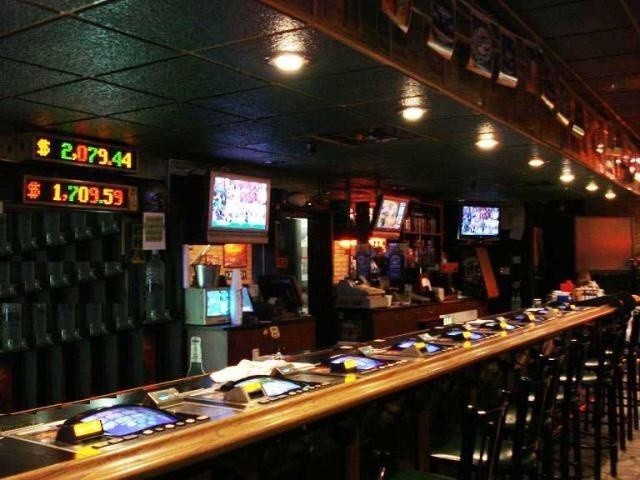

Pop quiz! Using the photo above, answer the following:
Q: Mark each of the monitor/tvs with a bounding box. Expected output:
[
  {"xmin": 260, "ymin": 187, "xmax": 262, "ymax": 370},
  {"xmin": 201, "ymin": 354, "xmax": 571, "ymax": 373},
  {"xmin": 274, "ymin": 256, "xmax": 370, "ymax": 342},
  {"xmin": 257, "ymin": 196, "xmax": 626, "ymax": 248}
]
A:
[
  {"xmin": 73, "ymin": 400, "xmax": 196, "ymax": 438},
  {"xmin": 328, "ymin": 352, "xmax": 391, "ymax": 372},
  {"xmin": 394, "ymin": 336, "xmax": 442, "ymax": 356},
  {"xmin": 233, "ymin": 375, "xmax": 303, "ymax": 398},
  {"xmin": 371, "ymin": 194, "xmax": 409, "ymax": 241},
  {"xmin": 256, "ymin": 275, "xmax": 303, "ymax": 310},
  {"xmin": 478, "ymin": 305, "xmax": 570, "ymax": 333},
  {"xmin": 445, "ymin": 326, "xmax": 488, "ymax": 341},
  {"xmin": 460, "ymin": 202, "xmax": 501, "ymax": 239},
  {"xmin": 206, "ymin": 169, "xmax": 273, "ymax": 244}
]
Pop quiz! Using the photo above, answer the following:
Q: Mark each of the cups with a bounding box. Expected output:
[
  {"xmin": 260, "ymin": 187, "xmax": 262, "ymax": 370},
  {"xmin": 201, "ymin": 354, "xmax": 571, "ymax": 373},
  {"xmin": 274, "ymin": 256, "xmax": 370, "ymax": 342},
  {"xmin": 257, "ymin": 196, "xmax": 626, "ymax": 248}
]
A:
[
  {"xmin": 533, "ymin": 299, "xmax": 542, "ymax": 306},
  {"xmin": 384, "ymin": 288, "xmax": 444, "ymax": 306},
  {"xmin": 229, "ymin": 268, "xmax": 243, "ymax": 325},
  {"xmin": 551, "ymin": 285, "xmax": 604, "ymax": 306}
]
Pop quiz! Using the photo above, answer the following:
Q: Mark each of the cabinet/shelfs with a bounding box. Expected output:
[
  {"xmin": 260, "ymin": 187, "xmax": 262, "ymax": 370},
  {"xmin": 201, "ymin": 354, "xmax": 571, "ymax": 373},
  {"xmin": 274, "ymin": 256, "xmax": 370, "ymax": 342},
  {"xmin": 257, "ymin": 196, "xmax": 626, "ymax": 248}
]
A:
[{"xmin": 307, "ymin": 206, "xmax": 526, "ymax": 339}]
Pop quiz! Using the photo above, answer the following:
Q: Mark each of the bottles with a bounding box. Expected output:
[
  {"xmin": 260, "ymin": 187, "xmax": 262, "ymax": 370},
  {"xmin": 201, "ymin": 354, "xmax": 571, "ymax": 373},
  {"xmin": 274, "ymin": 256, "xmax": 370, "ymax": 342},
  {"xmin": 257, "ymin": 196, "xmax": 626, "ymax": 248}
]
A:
[
  {"xmin": 510, "ymin": 292, "xmax": 522, "ymax": 310},
  {"xmin": 145, "ymin": 249, "xmax": 167, "ymax": 322},
  {"xmin": 186, "ymin": 336, "xmax": 205, "ymax": 378},
  {"xmin": 374, "ymin": 213, "xmax": 437, "ymax": 283}
]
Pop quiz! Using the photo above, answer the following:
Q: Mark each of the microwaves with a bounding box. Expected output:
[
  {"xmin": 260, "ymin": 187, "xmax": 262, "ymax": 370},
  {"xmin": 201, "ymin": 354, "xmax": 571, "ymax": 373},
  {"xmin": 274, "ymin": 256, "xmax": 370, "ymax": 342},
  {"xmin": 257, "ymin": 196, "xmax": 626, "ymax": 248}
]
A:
[{"xmin": 183, "ymin": 287, "xmax": 229, "ymax": 326}]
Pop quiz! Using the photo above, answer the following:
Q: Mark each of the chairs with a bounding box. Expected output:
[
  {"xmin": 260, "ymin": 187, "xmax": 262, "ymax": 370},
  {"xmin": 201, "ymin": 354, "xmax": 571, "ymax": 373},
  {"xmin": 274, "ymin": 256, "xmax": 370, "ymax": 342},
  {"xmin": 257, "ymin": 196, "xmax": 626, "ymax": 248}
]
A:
[{"xmin": 427, "ymin": 305, "xmax": 640, "ymax": 480}]
